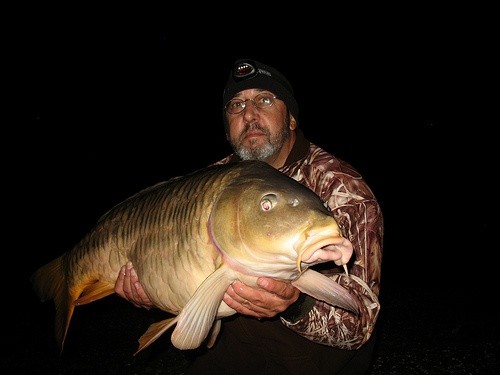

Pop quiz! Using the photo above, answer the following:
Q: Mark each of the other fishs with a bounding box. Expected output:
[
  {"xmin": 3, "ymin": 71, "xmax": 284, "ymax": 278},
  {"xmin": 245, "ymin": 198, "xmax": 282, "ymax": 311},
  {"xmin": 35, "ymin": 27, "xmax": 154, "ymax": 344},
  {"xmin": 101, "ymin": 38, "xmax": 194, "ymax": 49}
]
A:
[{"xmin": 36, "ymin": 158, "xmax": 363, "ymax": 353}]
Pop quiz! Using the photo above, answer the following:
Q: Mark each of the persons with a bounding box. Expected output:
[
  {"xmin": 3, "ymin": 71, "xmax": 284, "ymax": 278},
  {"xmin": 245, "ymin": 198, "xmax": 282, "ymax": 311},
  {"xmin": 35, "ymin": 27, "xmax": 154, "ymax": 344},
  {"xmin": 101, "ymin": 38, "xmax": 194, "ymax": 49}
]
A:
[{"xmin": 114, "ymin": 58, "xmax": 382, "ymax": 375}]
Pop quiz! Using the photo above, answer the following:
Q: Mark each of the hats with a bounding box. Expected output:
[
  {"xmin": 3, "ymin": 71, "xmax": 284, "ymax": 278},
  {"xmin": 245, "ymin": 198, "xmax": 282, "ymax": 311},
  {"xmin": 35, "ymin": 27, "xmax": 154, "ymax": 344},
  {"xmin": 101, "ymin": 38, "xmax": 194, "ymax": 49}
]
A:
[{"xmin": 219, "ymin": 60, "xmax": 301, "ymax": 123}]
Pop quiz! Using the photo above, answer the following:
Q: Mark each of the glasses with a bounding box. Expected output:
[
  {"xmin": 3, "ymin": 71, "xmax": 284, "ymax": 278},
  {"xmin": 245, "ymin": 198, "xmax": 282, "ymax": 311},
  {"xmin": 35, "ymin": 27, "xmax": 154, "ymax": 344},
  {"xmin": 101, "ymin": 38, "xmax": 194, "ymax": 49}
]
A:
[{"xmin": 222, "ymin": 92, "xmax": 283, "ymax": 116}]
